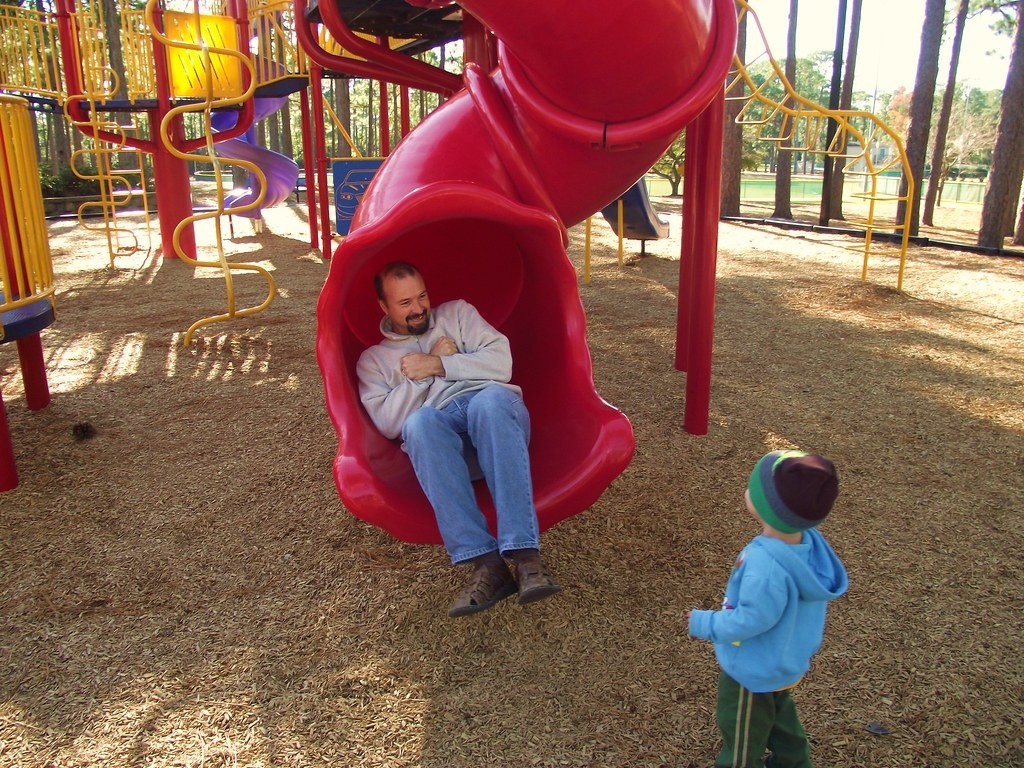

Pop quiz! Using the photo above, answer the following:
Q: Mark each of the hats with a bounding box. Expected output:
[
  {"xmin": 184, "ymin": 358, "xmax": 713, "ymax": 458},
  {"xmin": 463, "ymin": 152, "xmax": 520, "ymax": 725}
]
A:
[{"xmin": 749, "ymin": 449, "xmax": 838, "ymax": 534}]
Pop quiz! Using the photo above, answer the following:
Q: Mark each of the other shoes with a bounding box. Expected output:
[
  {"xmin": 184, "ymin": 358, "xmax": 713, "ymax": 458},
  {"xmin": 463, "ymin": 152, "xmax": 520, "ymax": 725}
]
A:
[{"xmin": 764, "ymin": 754, "xmax": 776, "ymax": 768}]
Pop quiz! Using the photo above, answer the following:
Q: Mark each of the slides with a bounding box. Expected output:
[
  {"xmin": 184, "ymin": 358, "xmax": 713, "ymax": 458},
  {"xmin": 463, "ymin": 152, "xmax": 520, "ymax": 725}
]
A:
[
  {"xmin": 205, "ymin": 0, "xmax": 299, "ymax": 220},
  {"xmin": 602, "ymin": 178, "xmax": 670, "ymax": 239},
  {"xmin": 318, "ymin": 0, "xmax": 736, "ymax": 545}
]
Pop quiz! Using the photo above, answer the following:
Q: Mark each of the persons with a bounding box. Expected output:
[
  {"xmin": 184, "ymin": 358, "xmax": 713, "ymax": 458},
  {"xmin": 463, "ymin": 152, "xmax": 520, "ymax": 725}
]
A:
[
  {"xmin": 356, "ymin": 262, "xmax": 561, "ymax": 616},
  {"xmin": 682, "ymin": 449, "xmax": 849, "ymax": 768}
]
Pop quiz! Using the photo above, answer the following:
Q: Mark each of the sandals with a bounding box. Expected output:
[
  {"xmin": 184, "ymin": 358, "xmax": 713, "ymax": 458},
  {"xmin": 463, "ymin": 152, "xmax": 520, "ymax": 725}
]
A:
[
  {"xmin": 448, "ymin": 548, "xmax": 517, "ymax": 616},
  {"xmin": 517, "ymin": 553, "xmax": 562, "ymax": 604}
]
